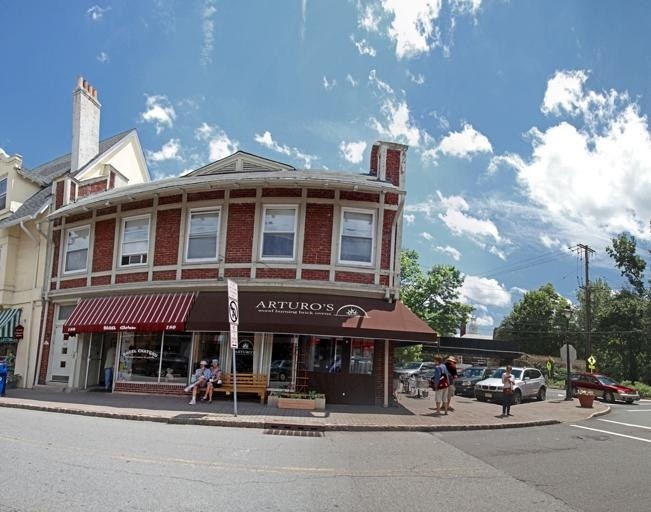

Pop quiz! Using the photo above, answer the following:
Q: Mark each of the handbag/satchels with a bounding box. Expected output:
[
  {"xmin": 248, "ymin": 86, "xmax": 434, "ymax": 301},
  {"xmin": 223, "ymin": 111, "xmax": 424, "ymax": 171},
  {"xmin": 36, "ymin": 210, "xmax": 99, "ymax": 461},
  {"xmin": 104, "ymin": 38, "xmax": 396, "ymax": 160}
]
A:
[{"xmin": 504, "ymin": 387, "xmax": 513, "ymax": 396}]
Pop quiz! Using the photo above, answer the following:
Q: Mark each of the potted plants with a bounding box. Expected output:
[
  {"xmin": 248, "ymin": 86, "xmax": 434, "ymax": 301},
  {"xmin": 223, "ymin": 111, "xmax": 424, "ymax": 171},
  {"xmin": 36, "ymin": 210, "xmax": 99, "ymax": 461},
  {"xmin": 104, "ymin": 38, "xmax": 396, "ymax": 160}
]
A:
[
  {"xmin": 3, "ymin": 351, "xmax": 22, "ymax": 388},
  {"xmin": 269, "ymin": 389, "xmax": 326, "ymax": 411},
  {"xmin": 574, "ymin": 388, "xmax": 596, "ymax": 407}
]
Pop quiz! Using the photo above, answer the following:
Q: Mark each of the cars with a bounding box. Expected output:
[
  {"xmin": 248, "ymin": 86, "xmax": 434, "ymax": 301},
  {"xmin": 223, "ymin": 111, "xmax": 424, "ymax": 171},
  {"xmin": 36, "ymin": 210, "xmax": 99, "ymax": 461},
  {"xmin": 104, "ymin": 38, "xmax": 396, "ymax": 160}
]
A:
[
  {"xmin": 391, "ymin": 361, "xmax": 438, "ymax": 384},
  {"xmin": 566, "ymin": 372, "xmax": 641, "ymax": 404},
  {"xmin": 453, "ymin": 366, "xmax": 494, "ymax": 398},
  {"xmin": 270, "ymin": 359, "xmax": 293, "ymax": 382},
  {"xmin": 132, "ymin": 352, "xmax": 189, "ymax": 378},
  {"xmin": 474, "ymin": 365, "xmax": 547, "ymax": 405}
]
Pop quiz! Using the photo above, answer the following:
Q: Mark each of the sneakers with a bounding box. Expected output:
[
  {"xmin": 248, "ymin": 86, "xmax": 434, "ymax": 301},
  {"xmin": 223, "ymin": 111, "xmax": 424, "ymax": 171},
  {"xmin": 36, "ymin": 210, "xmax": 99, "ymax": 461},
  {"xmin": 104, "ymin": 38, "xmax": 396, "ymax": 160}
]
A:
[
  {"xmin": 189, "ymin": 399, "xmax": 196, "ymax": 405},
  {"xmin": 183, "ymin": 387, "xmax": 191, "ymax": 393}
]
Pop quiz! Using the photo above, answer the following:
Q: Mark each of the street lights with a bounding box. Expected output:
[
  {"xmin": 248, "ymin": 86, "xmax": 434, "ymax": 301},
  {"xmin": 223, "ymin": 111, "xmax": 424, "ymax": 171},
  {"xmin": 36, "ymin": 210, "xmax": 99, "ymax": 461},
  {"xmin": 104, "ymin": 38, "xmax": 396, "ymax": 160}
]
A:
[{"xmin": 562, "ymin": 305, "xmax": 573, "ymax": 401}]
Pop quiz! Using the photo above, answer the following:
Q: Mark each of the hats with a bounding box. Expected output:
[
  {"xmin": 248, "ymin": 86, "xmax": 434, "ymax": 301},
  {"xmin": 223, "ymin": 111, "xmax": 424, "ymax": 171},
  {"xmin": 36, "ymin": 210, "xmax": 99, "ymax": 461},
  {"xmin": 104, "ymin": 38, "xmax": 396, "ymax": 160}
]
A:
[
  {"xmin": 199, "ymin": 360, "xmax": 208, "ymax": 365},
  {"xmin": 446, "ymin": 356, "xmax": 457, "ymax": 364}
]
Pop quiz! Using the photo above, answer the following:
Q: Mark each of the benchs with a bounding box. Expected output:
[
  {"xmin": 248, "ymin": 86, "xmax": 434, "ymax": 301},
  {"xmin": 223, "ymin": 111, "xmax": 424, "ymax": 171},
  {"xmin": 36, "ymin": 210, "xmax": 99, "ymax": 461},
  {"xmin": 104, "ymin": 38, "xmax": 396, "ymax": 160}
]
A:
[{"xmin": 196, "ymin": 374, "xmax": 266, "ymax": 404}]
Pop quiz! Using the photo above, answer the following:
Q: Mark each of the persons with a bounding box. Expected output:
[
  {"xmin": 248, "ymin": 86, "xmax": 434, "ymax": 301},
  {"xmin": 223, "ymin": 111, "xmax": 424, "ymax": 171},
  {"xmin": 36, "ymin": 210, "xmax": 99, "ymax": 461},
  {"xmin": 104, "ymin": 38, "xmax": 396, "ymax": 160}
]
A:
[
  {"xmin": 103, "ymin": 340, "xmax": 117, "ymax": 392},
  {"xmin": 498, "ymin": 365, "xmax": 516, "ymax": 416},
  {"xmin": 328, "ymin": 355, "xmax": 341, "ymax": 373},
  {"xmin": 184, "ymin": 359, "xmax": 222, "ymax": 405},
  {"xmin": 428, "ymin": 353, "xmax": 460, "ymax": 415}
]
